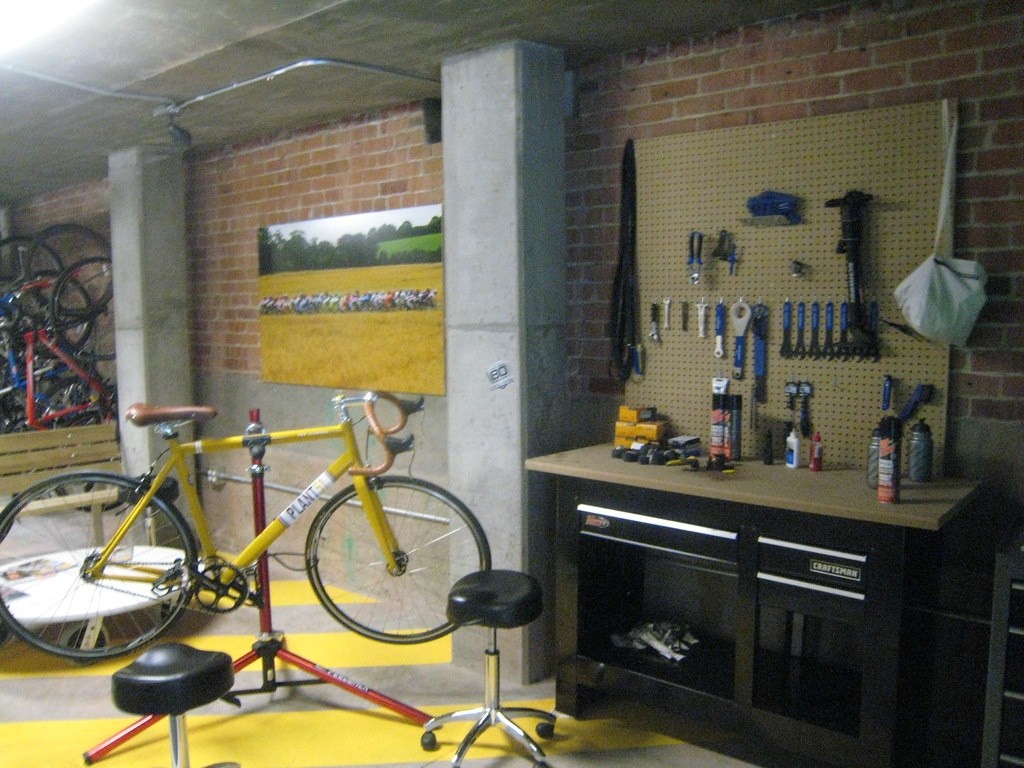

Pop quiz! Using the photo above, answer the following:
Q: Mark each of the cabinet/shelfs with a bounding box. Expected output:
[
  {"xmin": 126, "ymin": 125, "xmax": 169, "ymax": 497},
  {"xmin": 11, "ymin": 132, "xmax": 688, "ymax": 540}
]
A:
[
  {"xmin": 981, "ymin": 530, "xmax": 1024, "ymax": 767},
  {"xmin": 521, "ymin": 435, "xmax": 990, "ymax": 768}
]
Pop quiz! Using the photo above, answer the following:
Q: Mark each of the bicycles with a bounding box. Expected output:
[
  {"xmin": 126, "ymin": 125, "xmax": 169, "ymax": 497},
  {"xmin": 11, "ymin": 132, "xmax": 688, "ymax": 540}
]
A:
[
  {"xmin": 0, "ymin": 390, "xmax": 495, "ymax": 663},
  {"xmin": 0, "ymin": 222, "xmax": 116, "ymax": 437}
]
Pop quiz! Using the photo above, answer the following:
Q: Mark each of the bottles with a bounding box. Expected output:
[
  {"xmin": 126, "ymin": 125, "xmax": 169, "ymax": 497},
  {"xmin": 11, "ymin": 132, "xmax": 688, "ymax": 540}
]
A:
[
  {"xmin": 786, "ymin": 428, "xmax": 800, "ymax": 469},
  {"xmin": 710, "ymin": 377, "xmax": 731, "ymax": 462},
  {"xmin": 867, "ymin": 429, "xmax": 878, "ymax": 489},
  {"xmin": 809, "ymin": 432, "xmax": 824, "ymax": 471},
  {"xmin": 879, "ymin": 415, "xmax": 903, "ymax": 503},
  {"xmin": 731, "ymin": 392, "xmax": 745, "ymax": 459},
  {"xmin": 908, "ymin": 415, "xmax": 932, "ymax": 483}
]
